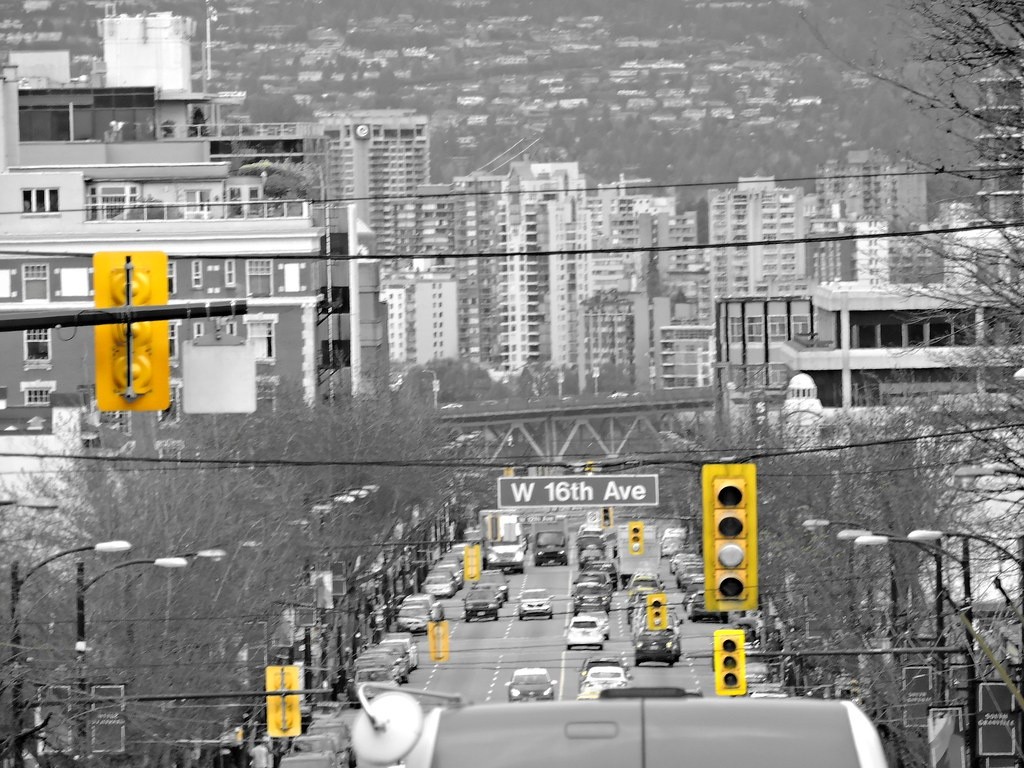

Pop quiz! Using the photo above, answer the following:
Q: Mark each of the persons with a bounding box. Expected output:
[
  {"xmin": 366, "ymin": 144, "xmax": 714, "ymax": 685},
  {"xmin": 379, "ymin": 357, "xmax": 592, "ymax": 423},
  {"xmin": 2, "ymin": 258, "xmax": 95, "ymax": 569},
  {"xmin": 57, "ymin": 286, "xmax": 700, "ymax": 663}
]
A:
[{"xmin": 222, "ymin": 707, "xmax": 312, "ymax": 768}]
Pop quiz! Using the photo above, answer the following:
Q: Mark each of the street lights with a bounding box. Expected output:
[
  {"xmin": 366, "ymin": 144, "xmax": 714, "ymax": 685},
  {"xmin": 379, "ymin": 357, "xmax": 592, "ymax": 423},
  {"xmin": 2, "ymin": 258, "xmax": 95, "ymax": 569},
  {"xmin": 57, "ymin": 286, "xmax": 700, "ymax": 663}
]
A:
[
  {"xmin": 834, "ymin": 527, "xmax": 950, "ymax": 706},
  {"xmin": 7, "ymin": 536, "xmax": 134, "ymax": 768},
  {"xmin": 853, "ymin": 532, "xmax": 981, "ymax": 768},
  {"xmin": 74, "ymin": 553, "xmax": 190, "ymax": 753},
  {"xmin": 904, "ymin": 528, "xmax": 1024, "ymax": 768},
  {"xmin": 163, "ymin": 547, "xmax": 227, "ymax": 639},
  {"xmin": 801, "ymin": 521, "xmax": 901, "ymax": 666},
  {"xmin": 422, "ymin": 370, "xmax": 438, "ymax": 410}
]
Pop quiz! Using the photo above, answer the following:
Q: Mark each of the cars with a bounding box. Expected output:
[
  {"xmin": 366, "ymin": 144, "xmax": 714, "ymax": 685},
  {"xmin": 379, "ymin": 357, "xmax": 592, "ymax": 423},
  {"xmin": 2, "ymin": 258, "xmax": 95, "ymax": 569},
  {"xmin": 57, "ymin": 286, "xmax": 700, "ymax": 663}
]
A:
[
  {"xmin": 515, "ymin": 588, "xmax": 557, "ymax": 621},
  {"xmin": 277, "ymin": 511, "xmax": 731, "ymax": 768},
  {"xmin": 504, "ymin": 667, "xmax": 558, "ymax": 702}
]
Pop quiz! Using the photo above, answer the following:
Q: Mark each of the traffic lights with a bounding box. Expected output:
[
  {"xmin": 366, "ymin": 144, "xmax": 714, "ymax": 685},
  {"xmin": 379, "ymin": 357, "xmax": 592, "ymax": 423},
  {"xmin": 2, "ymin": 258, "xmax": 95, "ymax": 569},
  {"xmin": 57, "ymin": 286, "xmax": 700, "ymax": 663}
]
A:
[
  {"xmin": 645, "ymin": 593, "xmax": 667, "ymax": 631},
  {"xmin": 628, "ymin": 520, "xmax": 645, "ymax": 556},
  {"xmin": 600, "ymin": 506, "xmax": 614, "ymax": 528},
  {"xmin": 713, "ymin": 629, "xmax": 747, "ymax": 697},
  {"xmin": 699, "ymin": 462, "xmax": 759, "ymax": 615}
]
